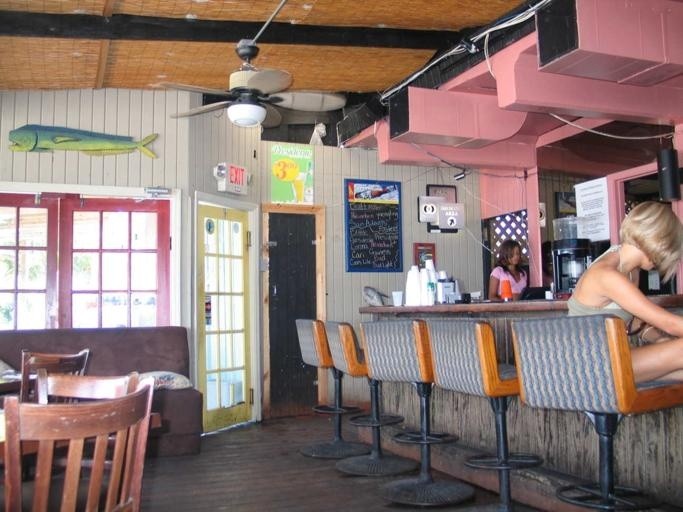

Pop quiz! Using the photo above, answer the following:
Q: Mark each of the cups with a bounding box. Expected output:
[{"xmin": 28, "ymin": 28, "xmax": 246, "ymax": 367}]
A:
[
  {"xmin": 404, "ymin": 259, "xmax": 462, "ymax": 307},
  {"xmin": 391, "ymin": 291, "xmax": 403, "ymax": 307}
]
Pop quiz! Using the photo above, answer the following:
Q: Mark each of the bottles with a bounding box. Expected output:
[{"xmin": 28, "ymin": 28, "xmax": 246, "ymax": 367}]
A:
[
  {"xmin": 354, "ymin": 186, "xmax": 396, "ymax": 201},
  {"xmin": 500, "ymin": 280, "xmax": 512, "ymax": 302}
]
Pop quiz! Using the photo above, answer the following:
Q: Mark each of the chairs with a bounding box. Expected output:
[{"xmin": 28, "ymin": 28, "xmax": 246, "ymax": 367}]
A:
[
  {"xmin": 359, "ymin": 318, "xmax": 476, "ymax": 508},
  {"xmin": 32, "ymin": 367, "xmax": 140, "ymax": 512},
  {"xmin": 323, "ymin": 316, "xmax": 418, "ymax": 477},
  {"xmin": 298, "ymin": 318, "xmax": 372, "ymax": 458},
  {"xmin": 511, "ymin": 312, "xmax": 681, "ymax": 512},
  {"xmin": 0, "ymin": 376, "xmax": 156, "ymax": 512},
  {"xmin": 17, "ymin": 347, "xmax": 88, "ymax": 405},
  {"xmin": 424, "ymin": 317, "xmax": 542, "ymax": 512}
]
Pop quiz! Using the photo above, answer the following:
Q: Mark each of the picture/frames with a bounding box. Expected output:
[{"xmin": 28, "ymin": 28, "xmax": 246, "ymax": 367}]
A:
[
  {"xmin": 425, "ymin": 183, "xmax": 459, "ymax": 234},
  {"xmin": 413, "ymin": 241, "xmax": 436, "ymax": 269}
]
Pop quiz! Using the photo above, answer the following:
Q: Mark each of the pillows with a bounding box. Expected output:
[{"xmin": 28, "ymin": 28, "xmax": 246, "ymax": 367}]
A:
[{"xmin": 140, "ymin": 371, "xmax": 189, "ymax": 390}]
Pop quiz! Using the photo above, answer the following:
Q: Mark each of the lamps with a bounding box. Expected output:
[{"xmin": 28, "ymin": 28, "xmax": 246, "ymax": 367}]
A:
[{"xmin": 222, "ymin": 95, "xmax": 266, "ymax": 126}]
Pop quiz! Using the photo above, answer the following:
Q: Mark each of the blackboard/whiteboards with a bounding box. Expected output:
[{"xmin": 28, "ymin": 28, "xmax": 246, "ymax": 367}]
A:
[{"xmin": 344, "ymin": 178, "xmax": 403, "ymax": 273}]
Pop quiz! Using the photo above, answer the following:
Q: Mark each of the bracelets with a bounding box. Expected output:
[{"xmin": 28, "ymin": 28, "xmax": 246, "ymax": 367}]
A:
[{"xmin": 639, "ymin": 325, "xmax": 655, "ymax": 342}]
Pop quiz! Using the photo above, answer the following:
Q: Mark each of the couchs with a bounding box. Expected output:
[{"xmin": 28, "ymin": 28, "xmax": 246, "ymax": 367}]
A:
[{"xmin": 0, "ymin": 327, "xmax": 204, "ymax": 460}]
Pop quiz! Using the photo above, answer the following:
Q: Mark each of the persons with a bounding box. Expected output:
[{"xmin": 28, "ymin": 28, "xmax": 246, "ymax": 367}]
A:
[
  {"xmin": 568, "ymin": 201, "xmax": 683, "ymax": 383},
  {"xmin": 488, "ymin": 239, "xmax": 527, "ymax": 301}
]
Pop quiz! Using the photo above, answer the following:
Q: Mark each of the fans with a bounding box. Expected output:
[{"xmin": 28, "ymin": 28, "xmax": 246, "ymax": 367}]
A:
[{"xmin": 151, "ymin": 40, "xmax": 345, "ymax": 128}]
